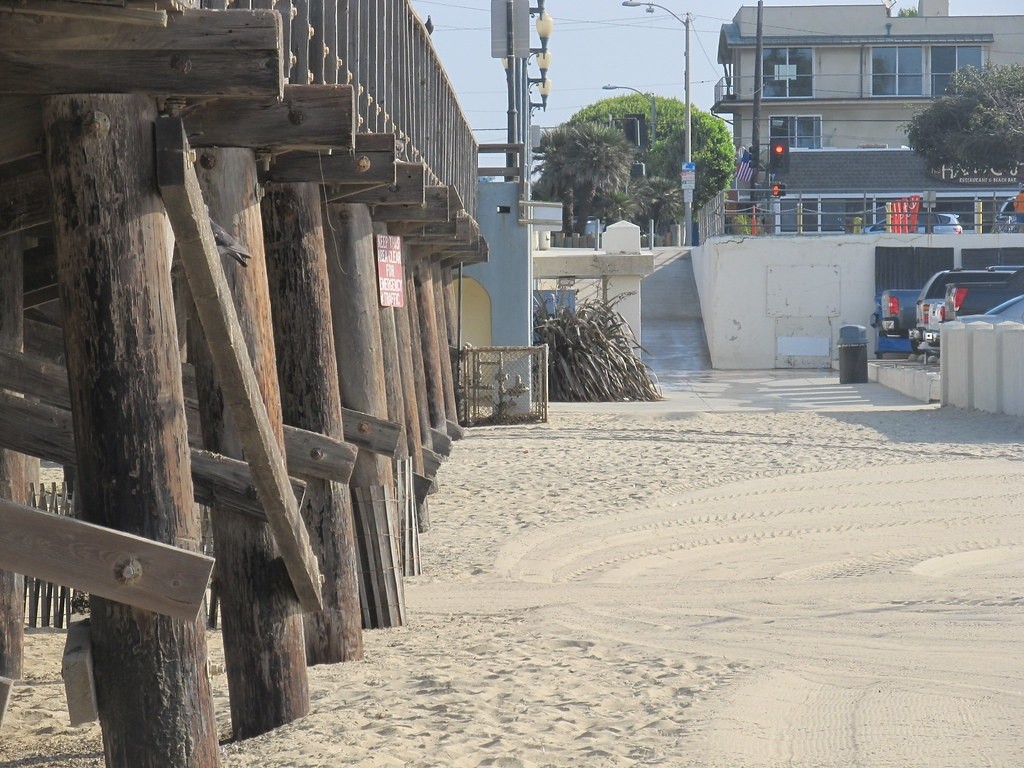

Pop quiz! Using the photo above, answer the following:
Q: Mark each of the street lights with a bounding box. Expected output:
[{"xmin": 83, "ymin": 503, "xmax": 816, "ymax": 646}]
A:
[
  {"xmin": 622, "ymin": 0, "xmax": 693, "ymax": 249},
  {"xmin": 602, "ymin": 85, "xmax": 654, "ymax": 250}
]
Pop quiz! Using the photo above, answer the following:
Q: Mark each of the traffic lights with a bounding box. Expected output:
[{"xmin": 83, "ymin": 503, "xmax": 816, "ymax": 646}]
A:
[
  {"xmin": 773, "ymin": 184, "xmax": 787, "ymax": 198},
  {"xmin": 748, "ymin": 146, "xmax": 760, "ymax": 169},
  {"xmin": 769, "ymin": 140, "xmax": 790, "ymax": 174}
]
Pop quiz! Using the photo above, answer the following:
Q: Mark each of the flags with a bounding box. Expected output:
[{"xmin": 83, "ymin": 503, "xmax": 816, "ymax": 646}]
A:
[{"xmin": 736, "ymin": 150, "xmax": 754, "ymax": 182}]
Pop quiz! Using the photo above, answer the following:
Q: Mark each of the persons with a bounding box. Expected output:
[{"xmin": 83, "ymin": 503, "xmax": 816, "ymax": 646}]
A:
[{"xmin": 1014, "ymin": 186, "xmax": 1024, "ymax": 232}]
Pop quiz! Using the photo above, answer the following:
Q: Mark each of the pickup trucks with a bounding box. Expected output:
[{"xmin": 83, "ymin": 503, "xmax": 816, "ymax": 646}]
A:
[
  {"xmin": 880, "ymin": 290, "xmax": 922, "ymax": 338},
  {"xmin": 943, "ymin": 268, "xmax": 1024, "ymax": 322}
]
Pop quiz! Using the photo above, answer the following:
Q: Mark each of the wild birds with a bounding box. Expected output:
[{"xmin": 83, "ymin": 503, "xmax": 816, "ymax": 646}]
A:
[{"xmin": 425, "ymin": 15, "xmax": 434, "ymax": 35}]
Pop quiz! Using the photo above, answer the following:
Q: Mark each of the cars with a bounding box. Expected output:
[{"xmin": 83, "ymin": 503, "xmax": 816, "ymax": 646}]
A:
[
  {"xmin": 862, "ymin": 212, "xmax": 963, "ymax": 236},
  {"xmin": 908, "ymin": 265, "xmax": 1024, "ymax": 355},
  {"xmin": 994, "ymin": 196, "xmax": 1017, "ymax": 231},
  {"xmin": 952, "ymin": 292, "xmax": 1024, "ymax": 326}
]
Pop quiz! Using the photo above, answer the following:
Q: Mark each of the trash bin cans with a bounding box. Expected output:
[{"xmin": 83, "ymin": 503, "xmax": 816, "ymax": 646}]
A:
[
  {"xmin": 837, "ymin": 325, "xmax": 868, "ymax": 384},
  {"xmin": 666, "ymin": 232, "xmax": 672, "ymax": 246},
  {"xmin": 539, "ymin": 230, "xmax": 550, "ymax": 250},
  {"xmin": 670, "ymin": 225, "xmax": 680, "ymax": 247},
  {"xmin": 842, "ymin": 216, "xmax": 855, "ymax": 234},
  {"xmin": 853, "ymin": 217, "xmax": 862, "ymax": 233},
  {"xmin": 533, "ymin": 231, "xmax": 540, "ymax": 251}
]
